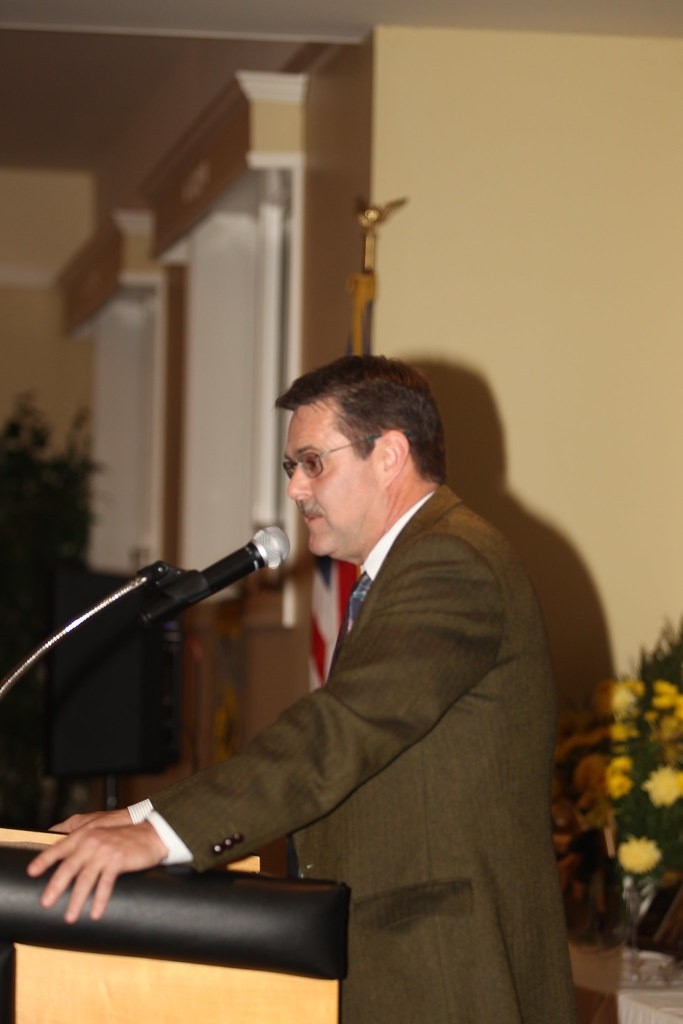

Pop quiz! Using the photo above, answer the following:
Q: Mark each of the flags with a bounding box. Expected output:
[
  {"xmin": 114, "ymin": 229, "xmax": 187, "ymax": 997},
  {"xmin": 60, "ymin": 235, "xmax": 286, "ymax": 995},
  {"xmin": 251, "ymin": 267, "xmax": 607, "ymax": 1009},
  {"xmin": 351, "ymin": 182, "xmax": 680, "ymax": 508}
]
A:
[{"xmin": 313, "ymin": 273, "xmax": 371, "ymax": 690}]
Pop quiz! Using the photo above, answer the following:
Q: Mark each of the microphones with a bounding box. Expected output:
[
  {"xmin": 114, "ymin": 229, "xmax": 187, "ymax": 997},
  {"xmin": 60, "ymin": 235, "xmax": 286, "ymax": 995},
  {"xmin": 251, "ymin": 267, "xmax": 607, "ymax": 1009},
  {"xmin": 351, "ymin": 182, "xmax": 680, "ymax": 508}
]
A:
[{"xmin": 129, "ymin": 525, "xmax": 291, "ymax": 632}]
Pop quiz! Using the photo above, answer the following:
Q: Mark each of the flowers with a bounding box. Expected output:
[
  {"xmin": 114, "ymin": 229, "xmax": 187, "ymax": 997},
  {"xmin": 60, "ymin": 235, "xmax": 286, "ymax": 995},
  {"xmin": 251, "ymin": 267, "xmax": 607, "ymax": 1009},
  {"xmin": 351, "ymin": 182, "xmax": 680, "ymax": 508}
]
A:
[{"xmin": 551, "ymin": 625, "xmax": 683, "ymax": 941}]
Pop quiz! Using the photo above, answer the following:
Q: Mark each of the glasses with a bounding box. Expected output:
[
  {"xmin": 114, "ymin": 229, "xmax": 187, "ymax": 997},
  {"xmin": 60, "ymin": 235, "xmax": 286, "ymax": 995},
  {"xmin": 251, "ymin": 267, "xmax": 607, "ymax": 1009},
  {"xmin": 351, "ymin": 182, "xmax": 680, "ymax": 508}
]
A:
[{"xmin": 282, "ymin": 429, "xmax": 418, "ymax": 480}]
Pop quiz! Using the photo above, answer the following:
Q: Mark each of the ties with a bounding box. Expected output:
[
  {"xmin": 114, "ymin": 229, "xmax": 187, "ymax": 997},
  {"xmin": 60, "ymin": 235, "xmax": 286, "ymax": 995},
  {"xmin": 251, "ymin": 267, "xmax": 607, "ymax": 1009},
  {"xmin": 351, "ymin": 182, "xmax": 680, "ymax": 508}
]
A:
[{"xmin": 343, "ymin": 572, "xmax": 372, "ymax": 636}]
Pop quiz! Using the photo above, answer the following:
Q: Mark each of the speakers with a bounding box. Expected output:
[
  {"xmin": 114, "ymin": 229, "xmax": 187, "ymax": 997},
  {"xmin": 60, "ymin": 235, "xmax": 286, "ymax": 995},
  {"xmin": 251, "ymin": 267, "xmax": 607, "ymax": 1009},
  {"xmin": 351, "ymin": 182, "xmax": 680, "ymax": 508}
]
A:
[{"xmin": 43, "ymin": 571, "xmax": 181, "ymax": 775}]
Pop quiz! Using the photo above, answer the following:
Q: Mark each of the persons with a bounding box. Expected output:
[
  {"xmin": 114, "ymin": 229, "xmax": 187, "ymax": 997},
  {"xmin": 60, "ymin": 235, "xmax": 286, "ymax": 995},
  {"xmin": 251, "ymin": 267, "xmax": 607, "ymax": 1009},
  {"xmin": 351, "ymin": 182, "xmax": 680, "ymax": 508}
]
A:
[{"xmin": 28, "ymin": 355, "xmax": 576, "ymax": 1024}]
